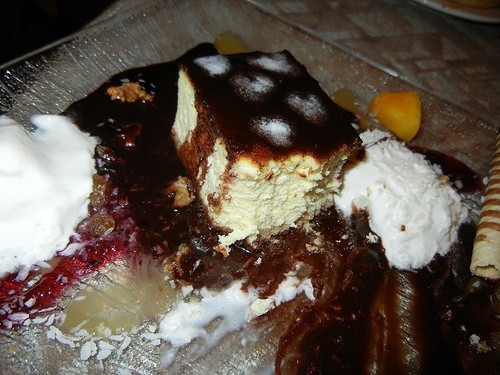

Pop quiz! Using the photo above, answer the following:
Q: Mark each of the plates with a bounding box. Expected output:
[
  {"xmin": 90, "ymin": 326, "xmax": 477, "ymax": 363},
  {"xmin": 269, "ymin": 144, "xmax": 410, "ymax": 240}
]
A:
[{"xmin": 0, "ymin": 0, "xmax": 500, "ymax": 375}]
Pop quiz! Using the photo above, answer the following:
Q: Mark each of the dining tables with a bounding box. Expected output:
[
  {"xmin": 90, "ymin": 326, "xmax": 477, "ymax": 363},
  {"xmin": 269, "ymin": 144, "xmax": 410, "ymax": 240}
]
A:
[{"xmin": 2, "ymin": 2, "xmax": 500, "ymax": 375}]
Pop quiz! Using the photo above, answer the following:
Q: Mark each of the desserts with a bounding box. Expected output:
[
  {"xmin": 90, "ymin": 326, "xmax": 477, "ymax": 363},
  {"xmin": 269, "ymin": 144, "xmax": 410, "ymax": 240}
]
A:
[{"xmin": 1, "ymin": 40, "xmax": 500, "ymax": 374}]
